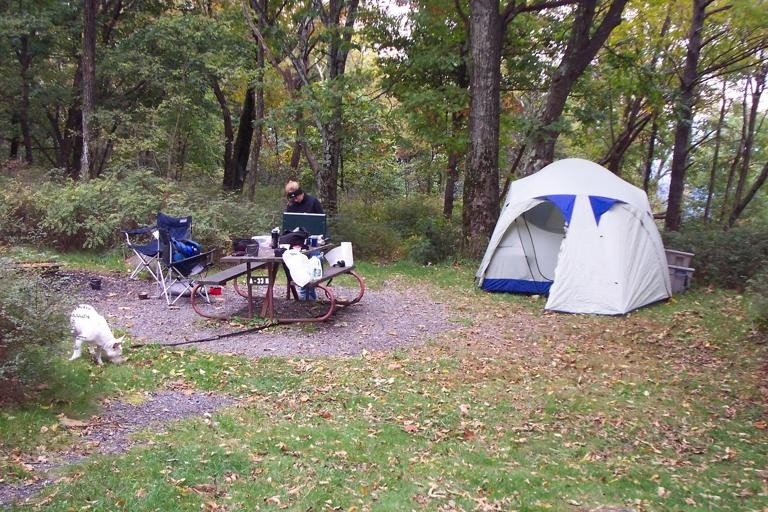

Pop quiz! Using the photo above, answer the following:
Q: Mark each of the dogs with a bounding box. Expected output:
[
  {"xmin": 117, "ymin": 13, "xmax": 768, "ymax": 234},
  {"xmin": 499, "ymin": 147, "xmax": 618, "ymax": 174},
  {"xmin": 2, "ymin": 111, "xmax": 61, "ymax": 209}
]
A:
[{"xmin": 69, "ymin": 304, "xmax": 126, "ymax": 367}]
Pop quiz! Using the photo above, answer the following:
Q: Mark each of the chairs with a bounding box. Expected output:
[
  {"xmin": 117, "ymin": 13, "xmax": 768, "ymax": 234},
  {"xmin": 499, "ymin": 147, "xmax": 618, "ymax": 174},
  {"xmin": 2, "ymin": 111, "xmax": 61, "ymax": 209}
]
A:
[{"xmin": 123, "ymin": 211, "xmax": 216, "ymax": 307}]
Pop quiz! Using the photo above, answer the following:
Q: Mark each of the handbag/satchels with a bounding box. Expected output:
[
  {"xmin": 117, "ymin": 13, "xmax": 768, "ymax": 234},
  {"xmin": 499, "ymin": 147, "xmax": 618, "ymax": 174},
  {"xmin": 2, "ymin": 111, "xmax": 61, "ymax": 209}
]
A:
[
  {"xmin": 278, "ymin": 227, "xmax": 309, "ymax": 251},
  {"xmin": 174, "ymin": 238, "xmax": 201, "ymax": 258},
  {"xmin": 234, "ymin": 238, "xmax": 258, "ymax": 256}
]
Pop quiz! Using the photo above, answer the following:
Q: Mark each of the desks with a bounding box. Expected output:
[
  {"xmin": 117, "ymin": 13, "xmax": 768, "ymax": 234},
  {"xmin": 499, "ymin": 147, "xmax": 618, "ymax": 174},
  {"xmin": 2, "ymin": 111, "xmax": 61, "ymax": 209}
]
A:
[{"xmin": 188, "ymin": 243, "xmax": 365, "ymax": 323}]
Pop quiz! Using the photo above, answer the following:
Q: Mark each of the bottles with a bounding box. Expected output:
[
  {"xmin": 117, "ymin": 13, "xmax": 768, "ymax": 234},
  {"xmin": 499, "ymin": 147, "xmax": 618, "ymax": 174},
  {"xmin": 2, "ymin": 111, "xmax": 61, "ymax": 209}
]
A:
[{"xmin": 271, "ymin": 229, "xmax": 278, "ymax": 249}]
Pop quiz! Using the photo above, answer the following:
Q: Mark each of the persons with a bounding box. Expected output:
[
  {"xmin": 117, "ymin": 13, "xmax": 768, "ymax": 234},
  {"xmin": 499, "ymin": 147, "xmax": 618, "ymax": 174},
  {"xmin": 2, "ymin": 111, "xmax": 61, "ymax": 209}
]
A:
[{"xmin": 284, "ymin": 180, "xmax": 326, "ymax": 302}]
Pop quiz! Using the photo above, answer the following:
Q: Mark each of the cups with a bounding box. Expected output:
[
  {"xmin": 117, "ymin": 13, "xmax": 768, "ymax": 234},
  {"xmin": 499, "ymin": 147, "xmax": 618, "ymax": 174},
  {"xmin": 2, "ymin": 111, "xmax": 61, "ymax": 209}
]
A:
[{"xmin": 308, "ymin": 236, "xmax": 318, "ymax": 247}]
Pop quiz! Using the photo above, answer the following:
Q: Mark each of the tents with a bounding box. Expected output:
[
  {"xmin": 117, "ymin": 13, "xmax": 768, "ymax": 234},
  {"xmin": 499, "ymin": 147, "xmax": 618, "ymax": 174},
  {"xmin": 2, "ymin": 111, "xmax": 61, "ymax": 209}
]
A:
[{"xmin": 473, "ymin": 157, "xmax": 673, "ymax": 316}]
